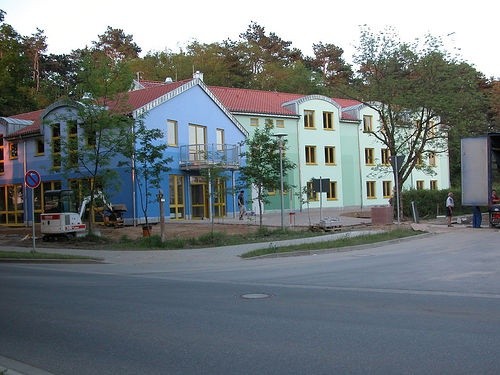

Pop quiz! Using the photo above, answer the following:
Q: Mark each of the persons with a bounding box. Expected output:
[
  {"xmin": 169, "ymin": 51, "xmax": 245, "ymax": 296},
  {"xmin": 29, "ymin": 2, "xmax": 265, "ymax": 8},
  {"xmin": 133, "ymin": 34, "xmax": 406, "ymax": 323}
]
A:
[
  {"xmin": 488, "ymin": 189, "xmax": 500, "ymax": 223},
  {"xmin": 446, "ymin": 192, "xmax": 454, "ymax": 227},
  {"xmin": 237, "ymin": 190, "xmax": 246, "ymax": 220},
  {"xmin": 471, "ymin": 205, "xmax": 483, "ymax": 228}
]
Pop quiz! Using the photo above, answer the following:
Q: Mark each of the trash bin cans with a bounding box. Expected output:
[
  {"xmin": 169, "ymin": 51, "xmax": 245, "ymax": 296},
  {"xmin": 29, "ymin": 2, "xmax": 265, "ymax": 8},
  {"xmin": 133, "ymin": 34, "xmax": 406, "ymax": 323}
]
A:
[{"xmin": 141, "ymin": 225, "xmax": 153, "ymax": 237}]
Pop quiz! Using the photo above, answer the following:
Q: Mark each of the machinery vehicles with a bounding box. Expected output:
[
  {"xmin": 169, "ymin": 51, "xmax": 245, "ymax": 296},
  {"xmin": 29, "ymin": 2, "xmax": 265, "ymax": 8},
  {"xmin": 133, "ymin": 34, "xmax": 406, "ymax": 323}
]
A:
[{"xmin": 40, "ymin": 189, "xmax": 125, "ymax": 243}]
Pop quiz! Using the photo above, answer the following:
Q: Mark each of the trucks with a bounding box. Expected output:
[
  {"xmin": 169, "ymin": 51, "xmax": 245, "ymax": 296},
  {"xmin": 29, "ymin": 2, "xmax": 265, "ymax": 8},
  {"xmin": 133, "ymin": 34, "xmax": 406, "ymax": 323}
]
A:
[{"xmin": 461, "ymin": 132, "xmax": 500, "ymax": 227}]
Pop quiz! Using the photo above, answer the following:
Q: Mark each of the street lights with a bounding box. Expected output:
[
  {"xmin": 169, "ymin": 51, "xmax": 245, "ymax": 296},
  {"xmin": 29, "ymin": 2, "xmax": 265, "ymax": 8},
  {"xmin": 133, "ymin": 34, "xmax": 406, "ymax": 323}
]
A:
[{"xmin": 270, "ymin": 133, "xmax": 288, "ymax": 234}]
